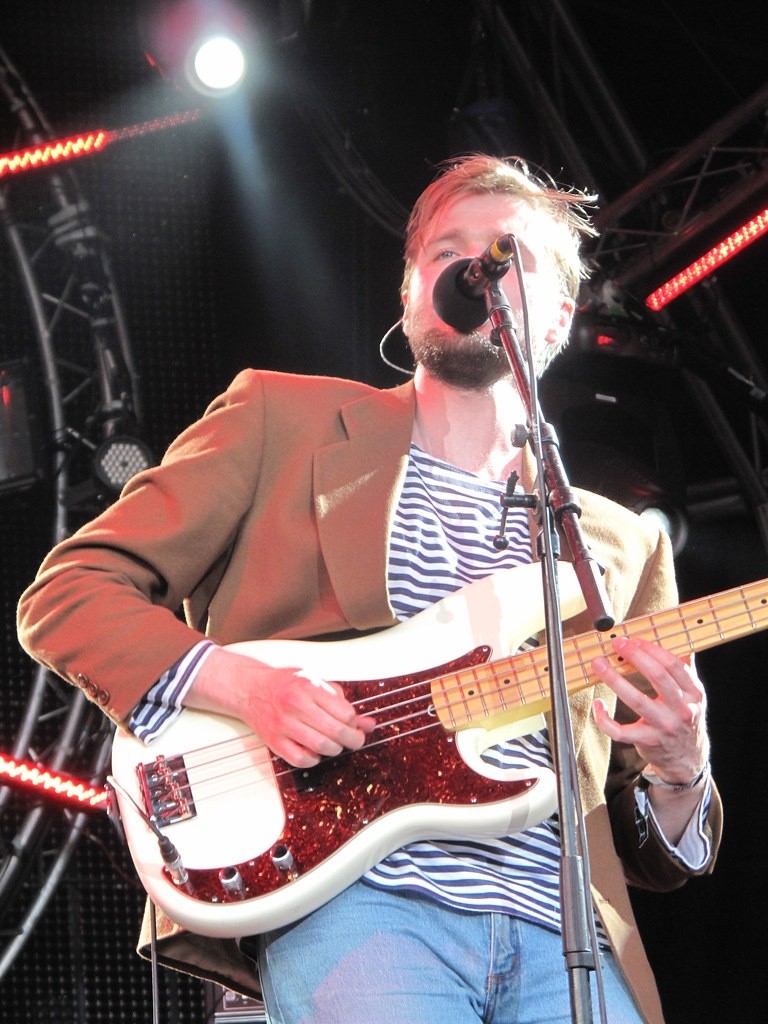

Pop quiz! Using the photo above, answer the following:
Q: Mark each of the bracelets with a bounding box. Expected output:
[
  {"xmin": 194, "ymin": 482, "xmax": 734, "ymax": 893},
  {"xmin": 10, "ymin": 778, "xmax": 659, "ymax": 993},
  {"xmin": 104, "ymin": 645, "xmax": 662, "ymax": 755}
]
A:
[{"xmin": 657, "ymin": 765, "xmax": 706, "ymax": 791}]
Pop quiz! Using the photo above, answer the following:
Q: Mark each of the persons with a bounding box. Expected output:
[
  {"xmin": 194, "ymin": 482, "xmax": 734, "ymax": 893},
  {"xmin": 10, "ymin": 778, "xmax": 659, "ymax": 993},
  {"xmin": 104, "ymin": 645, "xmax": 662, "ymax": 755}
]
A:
[{"xmin": 16, "ymin": 157, "xmax": 724, "ymax": 1024}]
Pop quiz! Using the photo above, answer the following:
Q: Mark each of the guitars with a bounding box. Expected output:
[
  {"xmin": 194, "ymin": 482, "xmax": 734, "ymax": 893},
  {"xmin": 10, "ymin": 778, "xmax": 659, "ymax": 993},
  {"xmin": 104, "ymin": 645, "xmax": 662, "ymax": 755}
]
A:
[{"xmin": 106, "ymin": 557, "xmax": 766, "ymax": 943}]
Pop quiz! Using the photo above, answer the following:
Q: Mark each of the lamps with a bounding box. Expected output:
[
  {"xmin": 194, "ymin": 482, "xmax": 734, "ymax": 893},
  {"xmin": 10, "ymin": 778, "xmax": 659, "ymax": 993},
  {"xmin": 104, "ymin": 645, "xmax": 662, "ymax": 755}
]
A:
[
  {"xmin": 93, "ymin": 434, "xmax": 161, "ymax": 493},
  {"xmin": 622, "ymin": 498, "xmax": 690, "ymax": 560}
]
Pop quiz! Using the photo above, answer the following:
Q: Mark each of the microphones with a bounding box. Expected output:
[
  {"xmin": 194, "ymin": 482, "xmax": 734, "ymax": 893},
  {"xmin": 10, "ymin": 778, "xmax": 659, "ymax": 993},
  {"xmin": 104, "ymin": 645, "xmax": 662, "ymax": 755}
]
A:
[{"xmin": 431, "ymin": 233, "xmax": 518, "ymax": 335}]
